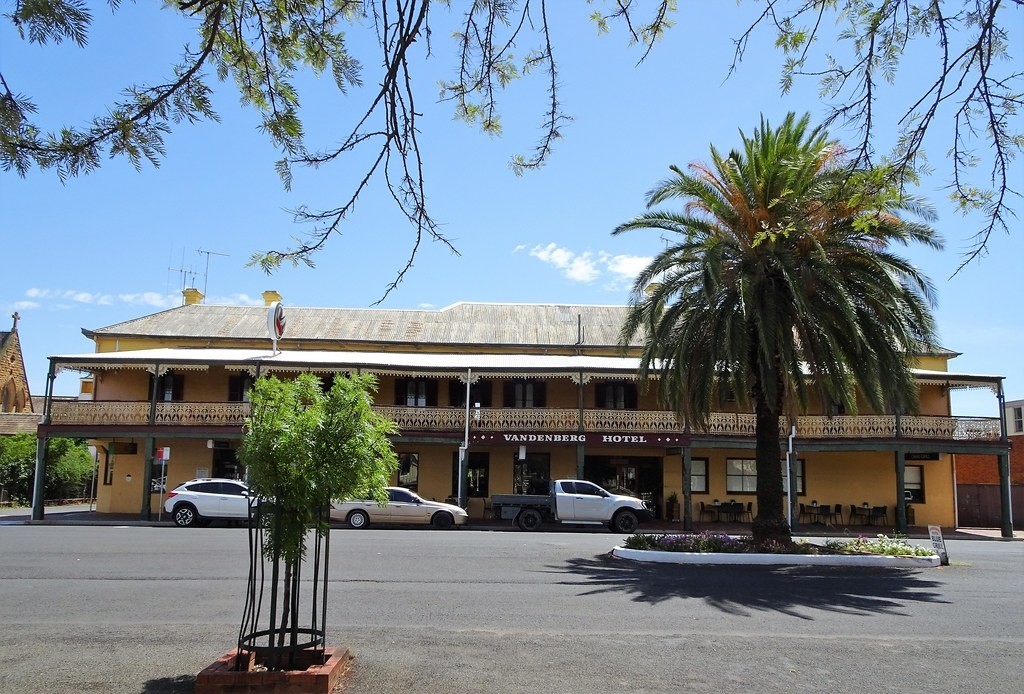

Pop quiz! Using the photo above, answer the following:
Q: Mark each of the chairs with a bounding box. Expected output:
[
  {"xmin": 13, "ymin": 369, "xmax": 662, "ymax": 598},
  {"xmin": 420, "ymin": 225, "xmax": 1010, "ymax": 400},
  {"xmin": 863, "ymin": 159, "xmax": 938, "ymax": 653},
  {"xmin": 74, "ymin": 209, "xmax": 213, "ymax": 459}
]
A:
[
  {"xmin": 849, "ymin": 504, "xmax": 867, "ymax": 528},
  {"xmin": 798, "ymin": 503, "xmax": 816, "ymax": 526},
  {"xmin": 718, "ymin": 503, "xmax": 732, "ymax": 524},
  {"xmin": 740, "ymin": 502, "xmax": 753, "ymax": 523},
  {"xmin": 699, "ymin": 501, "xmax": 715, "ymax": 524},
  {"xmin": 729, "ymin": 503, "xmax": 744, "ymax": 523},
  {"xmin": 867, "ymin": 506, "xmax": 889, "ymax": 528},
  {"xmin": 830, "ymin": 504, "xmax": 843, "ymax": 527},
  {"xmin": 814, "ymin": 504, "xmax": 830, "ymax": 527},
  {"xmin": 481, "ymin": 498, "xmax": 497, "ymax": 520},
  {"xmin": 433, "ymin": 497, "xmax": 470, "ymax": 506}
]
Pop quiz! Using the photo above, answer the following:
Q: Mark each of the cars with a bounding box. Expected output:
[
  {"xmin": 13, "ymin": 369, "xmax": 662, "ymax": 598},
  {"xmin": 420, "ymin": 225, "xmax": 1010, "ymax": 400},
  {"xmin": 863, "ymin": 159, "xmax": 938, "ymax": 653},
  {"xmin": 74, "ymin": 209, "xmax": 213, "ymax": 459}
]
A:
[{"xmin": 330, "ymin": 486, "xmax": 469, "ymax": 530}]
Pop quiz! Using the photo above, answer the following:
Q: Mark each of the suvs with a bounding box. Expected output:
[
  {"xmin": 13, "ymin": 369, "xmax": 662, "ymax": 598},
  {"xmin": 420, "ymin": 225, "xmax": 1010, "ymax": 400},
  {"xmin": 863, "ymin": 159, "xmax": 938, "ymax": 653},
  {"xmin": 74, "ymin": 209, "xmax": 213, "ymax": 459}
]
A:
[{"xmin": 164, "ymin": 477, "xmax": 276, "ymax": 528}]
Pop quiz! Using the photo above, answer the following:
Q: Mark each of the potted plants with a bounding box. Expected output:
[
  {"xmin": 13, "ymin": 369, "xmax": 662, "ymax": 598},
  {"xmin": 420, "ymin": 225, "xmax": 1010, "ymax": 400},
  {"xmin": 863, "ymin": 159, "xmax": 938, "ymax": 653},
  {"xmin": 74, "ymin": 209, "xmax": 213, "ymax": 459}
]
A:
[
  {"xmin": 863, "ymin": 502, "xmax": 869, "ymax": 508},
  {"xmin": 714, "ymin": 499, "xmax": 719, "ymax": 505},
  {"xmin": 448, "ymin": 495, "xmax": 453, "ymax": 499},
  {"xmin": 812, "ymin": 500, "xmax": 817, "ymax": 507},
  {"xmin": 730, "ymin": 499, "xmax": 736, "ymax": 505}
]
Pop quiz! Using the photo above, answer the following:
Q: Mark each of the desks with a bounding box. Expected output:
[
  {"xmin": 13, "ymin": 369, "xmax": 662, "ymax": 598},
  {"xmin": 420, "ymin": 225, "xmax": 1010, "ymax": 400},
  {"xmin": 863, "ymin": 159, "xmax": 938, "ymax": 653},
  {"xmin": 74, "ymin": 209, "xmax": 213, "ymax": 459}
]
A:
[
  {"xmin": 708, "ymin": 504, "xmax": 734, "ymax": 523},
  {"xmin": 443, "ymin": 501, "xmax": 458, "ymax": 503},
  {"xmin": 857, "ymin": 507, "xmax": 874, "ymax": 527},
  {"xmin": 806, "ymin": 505, "xmax": 824, "ymax": 526}
]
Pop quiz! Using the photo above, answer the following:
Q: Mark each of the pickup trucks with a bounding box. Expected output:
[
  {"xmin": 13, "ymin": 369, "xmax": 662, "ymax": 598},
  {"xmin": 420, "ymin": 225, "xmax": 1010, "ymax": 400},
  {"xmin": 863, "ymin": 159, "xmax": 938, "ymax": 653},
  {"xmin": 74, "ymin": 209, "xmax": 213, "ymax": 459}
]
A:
[{"xmin": 491, "ymin": 479, "xmax": 655, "ymax": 534}]
